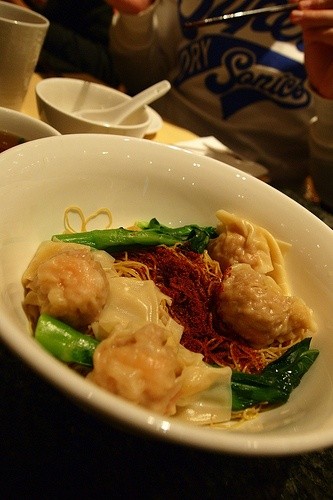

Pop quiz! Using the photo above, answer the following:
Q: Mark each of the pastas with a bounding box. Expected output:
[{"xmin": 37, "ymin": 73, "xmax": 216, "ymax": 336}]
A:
[{"xmin": 62, "ymin": 207, "xmax": 309, "ymax": 431}]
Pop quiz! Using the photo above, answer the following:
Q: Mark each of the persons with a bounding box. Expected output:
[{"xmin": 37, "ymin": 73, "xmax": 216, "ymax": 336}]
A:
[{"xmin": 107, "ymin": 0, "xmax": 333, "ymax": 211}]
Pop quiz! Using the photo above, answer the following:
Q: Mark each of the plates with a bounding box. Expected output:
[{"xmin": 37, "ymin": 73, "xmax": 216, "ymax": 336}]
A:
[{"xmin": 145, "ymin": 104, "xmax": 162, "ymax": 136}]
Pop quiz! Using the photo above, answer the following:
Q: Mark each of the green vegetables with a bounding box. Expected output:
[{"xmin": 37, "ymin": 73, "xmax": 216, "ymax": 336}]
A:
[
  {"xmin": 32, "ymin": 313, "xmax": 319, "ymax": 408},
  {"xmin": 52, "ymin": 216, "xmax": 218, "ymax": 254}
]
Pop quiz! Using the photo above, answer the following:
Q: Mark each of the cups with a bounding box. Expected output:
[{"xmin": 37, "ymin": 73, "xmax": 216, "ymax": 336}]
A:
[{"xmin": 0, "ymin": 0, "xmax": 50, "ymax": 113}]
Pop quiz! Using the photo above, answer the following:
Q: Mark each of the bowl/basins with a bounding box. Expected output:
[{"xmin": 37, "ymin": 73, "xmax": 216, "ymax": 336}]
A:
[
  {"xmin": 35, "ymin": 76, "xmax": 150, "ymax": 139},
  {"xmin": 0, "ymin": 107, "xmax": 62, "ymax": 152},
  {"xmin": 0, "ymin": 133, "xmax": 333, "ymax": 457}
]
